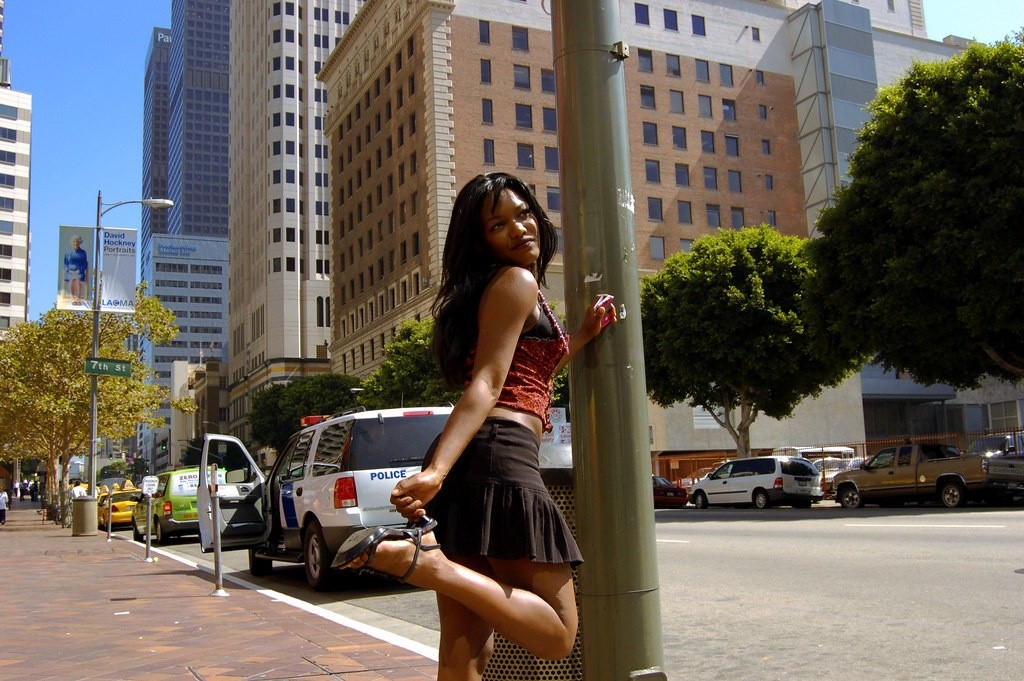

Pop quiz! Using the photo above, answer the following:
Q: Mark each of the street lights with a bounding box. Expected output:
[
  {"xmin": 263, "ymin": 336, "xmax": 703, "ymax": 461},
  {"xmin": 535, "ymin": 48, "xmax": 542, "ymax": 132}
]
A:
[{"xmin": 87, "ymin": 190, "xmax": 175, "ymax": 499}]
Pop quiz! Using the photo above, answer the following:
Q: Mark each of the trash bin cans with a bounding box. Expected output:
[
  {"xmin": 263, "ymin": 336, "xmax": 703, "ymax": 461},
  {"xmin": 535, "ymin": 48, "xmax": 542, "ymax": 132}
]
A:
[{"xmin": 72, "ymin": 495, "xmax": 99, "ymax": 536}]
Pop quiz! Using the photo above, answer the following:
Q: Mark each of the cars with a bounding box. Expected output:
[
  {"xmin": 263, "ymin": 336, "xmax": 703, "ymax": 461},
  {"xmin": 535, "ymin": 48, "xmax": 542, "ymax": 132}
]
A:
[
  {"xmin": 69, "ymin": 480, "xmax": 142, "ymax": 528},
  {"xmin": 132, "ymin": 464, "xmax": 229, "ymax": 546},
  {"xmin": 652, "ymin": 475, "xmax": 687, "ymax": 508},
  {"xmin": 672, "ymin": 432, "xmax": 1024, "ymax": 510}
]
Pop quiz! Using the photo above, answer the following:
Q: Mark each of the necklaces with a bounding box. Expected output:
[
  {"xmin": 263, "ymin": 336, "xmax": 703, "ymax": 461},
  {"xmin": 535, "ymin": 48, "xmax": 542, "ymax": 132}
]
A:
[{"xmin": 538, "ymin": 290, "xmax": 569, "ymax": 354}]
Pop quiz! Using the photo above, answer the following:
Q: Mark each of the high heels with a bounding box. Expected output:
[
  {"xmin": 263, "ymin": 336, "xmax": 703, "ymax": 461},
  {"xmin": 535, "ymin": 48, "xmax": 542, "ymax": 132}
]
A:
[{"xmin": 331, "ymin": 494, "xmax": 442, "ymax": 584}]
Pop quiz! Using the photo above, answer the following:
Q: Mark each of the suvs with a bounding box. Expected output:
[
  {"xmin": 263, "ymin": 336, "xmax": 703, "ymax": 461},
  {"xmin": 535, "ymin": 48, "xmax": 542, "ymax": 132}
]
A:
[{"xmin": 195, "ymin": 403, "xmax": 456, "ymax": 590}]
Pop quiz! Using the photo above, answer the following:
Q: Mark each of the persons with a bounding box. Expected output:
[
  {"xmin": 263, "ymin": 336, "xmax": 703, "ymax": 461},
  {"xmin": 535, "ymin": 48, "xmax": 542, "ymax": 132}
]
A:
[
  {"xmin": 12, "ymin": 479, "xmax": 39, "ymax": 502},
  {"xmin": 64, "ymin": 235, "xmax": 89, "ymax": 305},
  {"xmin": 332, "ymin": 171, "xmax": 618, "ymax": 681},
  {"xmin": 0, "ymin": 486, "xmax": 9, "ymax": 525},
  {"xmin": 69, "ymin": 479, "xmax": 88, "ymax": 499}
]
